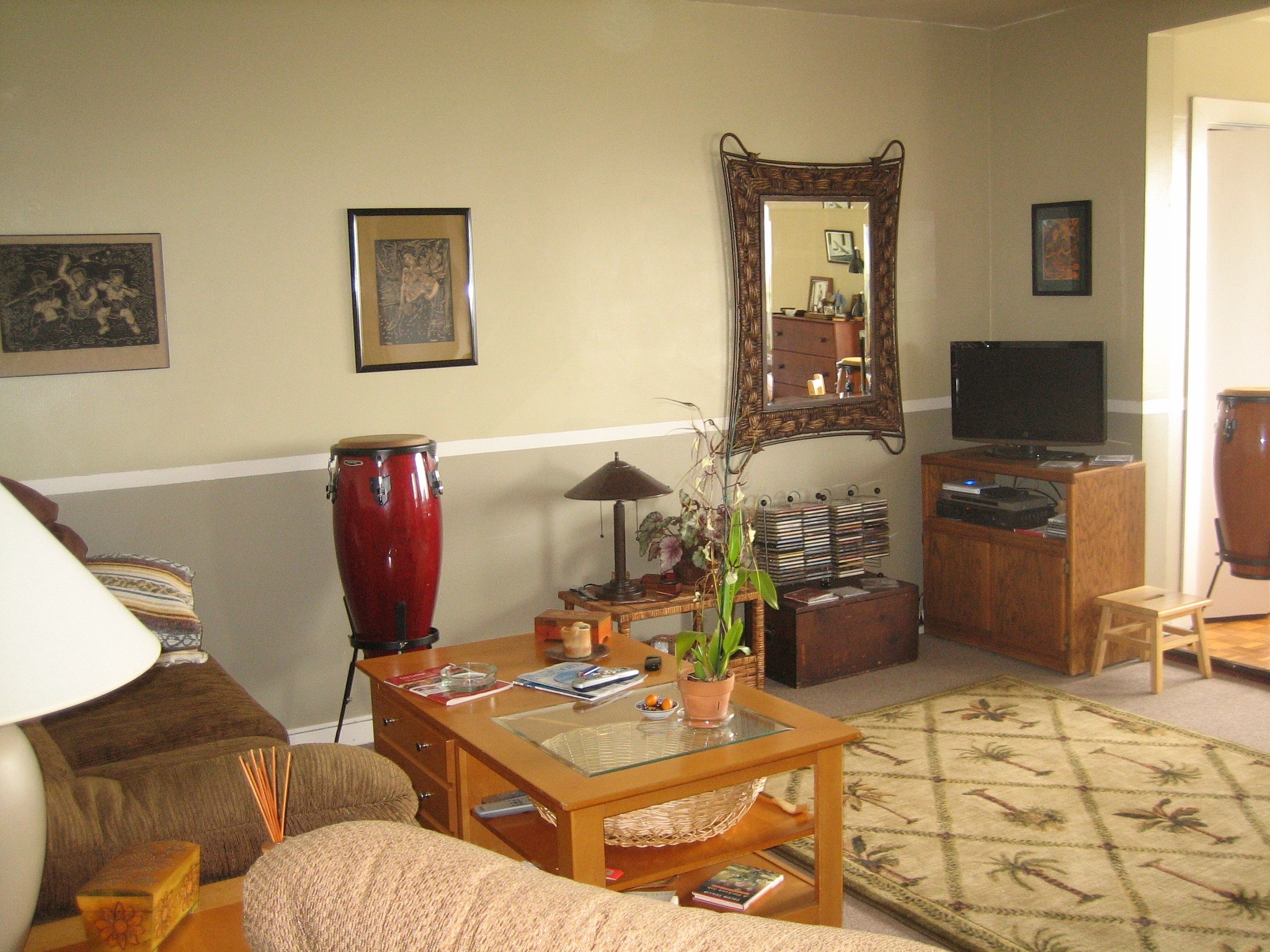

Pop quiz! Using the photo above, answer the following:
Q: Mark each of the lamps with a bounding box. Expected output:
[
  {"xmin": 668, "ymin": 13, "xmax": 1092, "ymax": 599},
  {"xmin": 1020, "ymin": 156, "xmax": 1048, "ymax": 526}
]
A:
[
  {"xmin": 566, "ymin": 453, "xmax": 675, "ymax": 605},
  {"xmin": 847, "ymin": 250, "xmax": 865, "ymax": 275},
  {"xmin": 0, "ymin": 489, "xmax": 161, "ymax": 951}
]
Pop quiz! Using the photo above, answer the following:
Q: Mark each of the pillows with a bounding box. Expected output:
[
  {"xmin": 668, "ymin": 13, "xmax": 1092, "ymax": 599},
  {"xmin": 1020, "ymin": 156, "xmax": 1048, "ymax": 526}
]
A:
[{"xmin": 80, "ymin": 551, "xmax": 208, "ymax": 666}]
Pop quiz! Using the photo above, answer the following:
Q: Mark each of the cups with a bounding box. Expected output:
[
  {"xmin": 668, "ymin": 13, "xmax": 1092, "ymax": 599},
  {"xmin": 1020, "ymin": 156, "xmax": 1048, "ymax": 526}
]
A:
[
  {"xmin": 852, "ymin": 294, "xmax": 864, "ymax": 318},
  {"xmin": 561, "ymin": 622, "xmax": 591, "ymax": 658},
  {"xmin": 644, "ymin": 655, "xmax": 661, "ymax": 671}
]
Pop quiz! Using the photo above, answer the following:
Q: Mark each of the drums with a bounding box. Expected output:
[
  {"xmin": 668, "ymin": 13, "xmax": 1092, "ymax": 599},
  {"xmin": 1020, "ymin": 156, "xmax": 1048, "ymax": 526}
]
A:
[
  {"xmin": 1211, "ymin": 387, "xmax": 1270, "ymax": 581},
  {"xmin": 329, "ymin": 433, "xmax": 444, "ymax": 660}
]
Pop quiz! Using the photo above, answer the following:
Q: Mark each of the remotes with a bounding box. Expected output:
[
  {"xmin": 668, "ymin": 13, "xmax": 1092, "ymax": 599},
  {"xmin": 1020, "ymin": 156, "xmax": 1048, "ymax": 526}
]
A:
[
  {"xmin": 474, "ymin": 789, "xmax": 536, "ymax": 818},
  {"xmin": 571, "ymin": 667, "xmax": 639, "ymax": 693}
]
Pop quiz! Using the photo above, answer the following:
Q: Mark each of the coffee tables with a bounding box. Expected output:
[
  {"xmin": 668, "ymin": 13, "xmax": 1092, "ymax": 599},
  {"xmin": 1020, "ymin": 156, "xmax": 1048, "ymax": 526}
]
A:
[{"xmin": 354, "ymin": 634, "xmax": 859, "ymax": 927}]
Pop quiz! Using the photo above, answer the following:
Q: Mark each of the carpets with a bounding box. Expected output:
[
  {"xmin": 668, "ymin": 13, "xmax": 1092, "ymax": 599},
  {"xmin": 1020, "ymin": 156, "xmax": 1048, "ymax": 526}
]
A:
[{"xmin": 744, "ymin": 676, "xmax": 1270, "ymax": 951}]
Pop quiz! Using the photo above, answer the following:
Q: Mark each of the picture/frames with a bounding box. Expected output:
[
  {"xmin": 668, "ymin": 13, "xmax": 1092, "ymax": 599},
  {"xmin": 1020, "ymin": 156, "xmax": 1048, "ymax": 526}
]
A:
[
  {"xmin": 824, "ymin": 230, "xmax": 854, "ymax": 264},
  {"xmin": 806, "ymin": 276, "xmax": 833, "ymax": 311},
  {"xmin": 346, "ymin": 206, "xmax": 480, "ymax": 373},
  {"xmin": 1030, "ymin": 199, "xmax": 1093, "ymax": 298}
]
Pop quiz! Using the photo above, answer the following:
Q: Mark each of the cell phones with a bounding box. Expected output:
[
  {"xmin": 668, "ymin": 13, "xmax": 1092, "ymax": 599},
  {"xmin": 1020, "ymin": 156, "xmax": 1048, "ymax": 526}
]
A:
[{"xmin": 645, "ymin": 656, "xmax": 661, "ymax": 670}]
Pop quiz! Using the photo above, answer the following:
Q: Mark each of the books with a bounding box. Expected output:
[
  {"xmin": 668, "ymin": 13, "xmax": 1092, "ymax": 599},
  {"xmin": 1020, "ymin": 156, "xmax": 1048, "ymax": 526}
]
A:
[
  {"xmin": 692, "ymin": 865, "xmax": 784, "ymax": 911},
  {"xmin": 383, "ymin": 662, "xmax": 514, "ymax": 706},
  {"xmin": 512, "ymin": 660, "xmax": 648, "ymax": 700}
]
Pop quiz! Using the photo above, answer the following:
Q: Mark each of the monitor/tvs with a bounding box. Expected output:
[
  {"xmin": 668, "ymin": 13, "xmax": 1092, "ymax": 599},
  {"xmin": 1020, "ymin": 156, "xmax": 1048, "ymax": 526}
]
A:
[{"xmin": 950, "ymin": 340, "xmax": 1108, "ymax": 462}]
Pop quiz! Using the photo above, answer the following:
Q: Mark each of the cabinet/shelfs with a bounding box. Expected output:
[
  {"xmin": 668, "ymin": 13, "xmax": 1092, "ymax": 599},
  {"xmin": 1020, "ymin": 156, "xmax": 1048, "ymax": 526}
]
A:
[
  {"xmin": 758, "ymin": 569, "xmax": 922, "ymax": 688},
  {"xmin": 917, "ymin": 444, "xmax": 1144, "ymax": 676},
  {"xmin": 770, "ymin": 312, "xmax": 864, "ymax": 398}
]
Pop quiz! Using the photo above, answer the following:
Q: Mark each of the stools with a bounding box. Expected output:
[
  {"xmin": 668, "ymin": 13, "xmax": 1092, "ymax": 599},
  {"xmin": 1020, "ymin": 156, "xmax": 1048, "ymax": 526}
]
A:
[{"xmin": 1092, "ymin": 586, "xmax": 1212, "ymax": 695}]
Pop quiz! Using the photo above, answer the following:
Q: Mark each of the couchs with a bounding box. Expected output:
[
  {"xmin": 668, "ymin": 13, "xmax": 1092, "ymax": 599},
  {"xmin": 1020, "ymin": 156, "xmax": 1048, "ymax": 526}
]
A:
[{"xmin": 0, "ymin": 477, "xmax": 960, "ymax": 951}]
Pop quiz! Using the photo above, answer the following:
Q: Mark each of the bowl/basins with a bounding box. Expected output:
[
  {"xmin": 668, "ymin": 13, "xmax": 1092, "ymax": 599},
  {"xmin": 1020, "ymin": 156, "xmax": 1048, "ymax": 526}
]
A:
[
  {"xmin": 635, "ymin": 699, "xmax": 679, "ymax": 720},
  {"xmin": 780, "ymin": 307, "xmax": 795, "ymax": 316},
  {"xmin": 785, "ymin": 309, "xmax": 796, "ymax": 316}
]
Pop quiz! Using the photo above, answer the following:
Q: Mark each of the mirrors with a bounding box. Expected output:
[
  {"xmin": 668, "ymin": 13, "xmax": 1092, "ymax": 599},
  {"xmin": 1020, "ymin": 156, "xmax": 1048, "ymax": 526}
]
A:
[{"xmin": 715, "ymin": 131, "xmax": 906, "ymax": 473}]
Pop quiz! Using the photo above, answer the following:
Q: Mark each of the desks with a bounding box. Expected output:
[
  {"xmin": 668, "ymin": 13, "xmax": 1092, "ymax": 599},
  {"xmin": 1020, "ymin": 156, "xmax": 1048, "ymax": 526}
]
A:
[{"xmin": 556, "ymin": 577, "xmax": 767, "ymax": 686}]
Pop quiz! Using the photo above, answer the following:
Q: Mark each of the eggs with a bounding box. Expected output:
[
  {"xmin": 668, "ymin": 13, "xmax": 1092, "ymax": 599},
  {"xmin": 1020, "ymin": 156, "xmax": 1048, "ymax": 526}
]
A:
[
  {"xmin": 661, "ymin": 698, "xmax": 673, "ymax": 711},
  {"xmin": 645, "ymin": 694, "xmax": 658, "ymax": 706}
]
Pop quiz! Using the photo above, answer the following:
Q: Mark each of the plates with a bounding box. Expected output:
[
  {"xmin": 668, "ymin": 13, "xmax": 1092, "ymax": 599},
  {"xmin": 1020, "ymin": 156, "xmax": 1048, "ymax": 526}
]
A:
[{"xmin": 543, "ymin": 644, "xmax": 612, "ymax": 662}]
